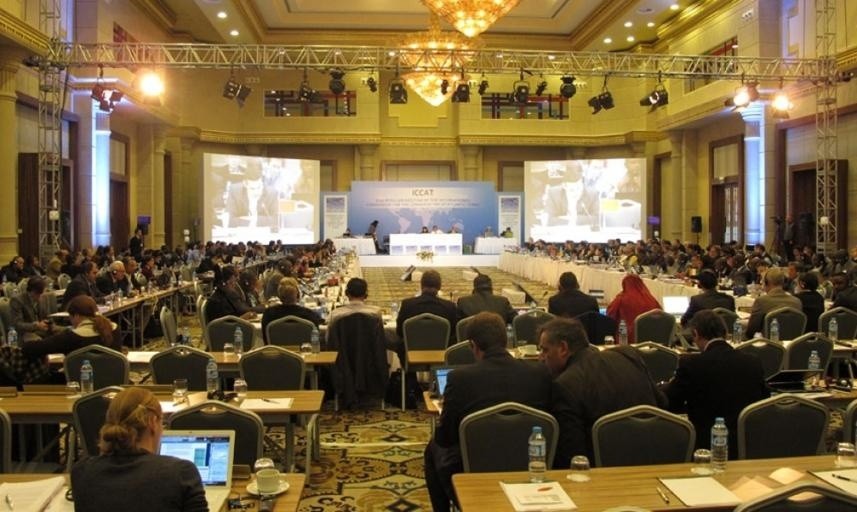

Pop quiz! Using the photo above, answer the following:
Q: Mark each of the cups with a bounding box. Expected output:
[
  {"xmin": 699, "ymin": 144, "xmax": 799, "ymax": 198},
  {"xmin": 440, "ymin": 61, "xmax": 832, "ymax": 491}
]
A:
[
  {"xmin": 567, "ymin": 453, "xmax": 593, "ymax": 482},
  {"xmin": 301, "ymin": 341, "xmax": 311, "ymax": 356},
  {"xmin": 234, "ymin": 377, "xmax": 249, "ymax": 397},
  {"xmin": 692, "ymin": 448, "xmax": 714, "ymax": 477},
  {"xmin": 173, "ymin": 378, "xmax": 188, "ymax": 396},
  {"xmin": 255, "ymin": 468, "xmax": 287, "ymax": 493},
  {"xmin": 830, "ymin": 440, "xmax": 856, "ymax": 467},
  {"xmin": 603, "ymin": 333, "xmax": 616, "ymax": 348},
  {"xmin": 65, "ymin": 381, "xmax": 80, "ymax": 398},
  {"xmin": 223, "ymin": 342, "xmax": 234, "ymax": 355}
]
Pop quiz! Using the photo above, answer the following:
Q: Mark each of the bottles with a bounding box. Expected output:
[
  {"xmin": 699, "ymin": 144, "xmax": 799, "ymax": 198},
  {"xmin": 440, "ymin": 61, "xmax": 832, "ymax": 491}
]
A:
[
  {"xmin": 807, "ymin": 349, "xmax": 820, "ymax": 369},
  {"xmin": 108, "ymin": 260, "xmax": 180, "ymax": 307},
  {"xmin": 505, "ymin": 324, "xmax": 515, "ymax": 348},
  {"xmin": 206, "ymin": 359, "xmax": 219, "ymax": 392},
  {"xmin": 310, "ymin": 326, "xmax": 320, "ymax": 355},
  {"xmin": 527, "ymin": 426, "xmax": 549, "ymax": 484},
  {"xmin": 7, "ymin": 326, "xmax": 18, "ymax": 348},
  {"xmin": 709, "ymin": 417, "xmax": 730, "ymax": 473},
  {"xmin": 233, "ymin": 325, "xmax": 244, "ymax": 355},
  {"xmin": 770, "ymin": 317, "xmax": 781, "ymax": 343},
  {"xmin": 79, "ymin": 359, "xmax": 94, "ymax": 396},
  {"xmin": 732, "ymin": 317, "xmax": 742, "ymax": 343},
  {"xmin": 617, "ymin": 319, "xmax": 628, "ymax": 344},
  {"xmin": 828, "ymin": 316, "xmax": 840, "ymax": 345}
]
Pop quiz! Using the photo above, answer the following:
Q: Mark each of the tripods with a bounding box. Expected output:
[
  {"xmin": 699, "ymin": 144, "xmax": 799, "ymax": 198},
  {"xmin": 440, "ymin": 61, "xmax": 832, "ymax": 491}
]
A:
[{"xmin": 769, "ymin": 227, "xmax": 784, "ymax": 255}]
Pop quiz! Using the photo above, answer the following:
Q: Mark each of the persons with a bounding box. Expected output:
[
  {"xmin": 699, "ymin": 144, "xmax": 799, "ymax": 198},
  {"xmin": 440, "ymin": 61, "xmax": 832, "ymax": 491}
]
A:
[
  {"xmin": 457, "ymin": 273, "xmax": 519, "ymax": 327},
  {"xmin": 424, "ymin": 311, "xmax": 771, "ymax": 512},
  {"xmin": 208, "ymin": 158, "xmax": 318, "ymax": 227},
  {"xmin": 530, "ymin": 160, "xmax": 643, "ymax": 226},
  {"xmin": 524, "ymin": 238, "xmax": 857, "ymax": 382},
  {"xmin": 2, "ymin": 218, "xmax": 382, "ymax": 386},
  {"xmin": 395, "ymin": 268, "xmax": 457, "ymax": 401},
  {"xmin": 421, "ymin": 224, "xmax": 513, "ymax": 237},
  {"xmin": 70, "ymin": 387, "xmax": 210, "ymax": 512}
]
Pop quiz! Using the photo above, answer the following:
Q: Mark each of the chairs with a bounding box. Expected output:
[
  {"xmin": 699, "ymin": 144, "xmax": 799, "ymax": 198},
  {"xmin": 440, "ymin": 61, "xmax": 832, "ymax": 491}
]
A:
[
  {"xmin": 457, "ymin": 400, "xmax": 562, "ymax": 474},
  {"xmin": 731, "ymin": 480, "xmax": 857, "ymax": 511},
  {"xmin": 843, "ymin": 400, "xmax": 857, "ymax": 453},
  {"xmin": 236, "ymin": 344, "xmax": 307, "ymax": 472},
  {"xmin": 167, "ymin": 400, "xmax": 266, "ymax": 469},
  {"xmin": 593, "ymin": 403, "xmax": 699, "ymax": 467},
  {"xmin": 72, "ymin": 386, "xmax": 125, "ymax": 460},
  {"xmin": 738, "ymin": 391, "xmax": 832, "ymax": 460},
  {"xmin": 0, "ymin": 408, "xmax": 15, "ymax": 473}
]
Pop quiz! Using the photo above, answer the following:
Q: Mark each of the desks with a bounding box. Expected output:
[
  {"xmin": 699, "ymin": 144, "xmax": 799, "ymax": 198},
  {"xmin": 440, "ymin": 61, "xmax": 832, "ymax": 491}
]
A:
[
  {"xmin": 499, "ymin": 249, "xmax": 753, "ymax": 315},
  {"xmin": 330, "ymin": 237, "xmax": 377, "ymax": 255},
  {"xmin": 0, "ymin": 470, "xmax": 309, "ymax": 512},
  {"xmin": 0, "ymin": 388, "xmax": 326, "ymax": 489},
  {"xmin": 472, "ymin": 236, "xmax": 518, "ymax": 255},
  {"xmin": 449, "ymin": 452, "xmax": 855, "ymax": 511}
]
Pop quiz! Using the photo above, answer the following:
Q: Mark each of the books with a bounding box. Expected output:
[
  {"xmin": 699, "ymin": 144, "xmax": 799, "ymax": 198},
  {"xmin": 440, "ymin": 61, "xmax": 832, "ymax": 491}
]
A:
[{"xmin": 1, "ymin": 477, "xmax": 66, "ymax": 512}]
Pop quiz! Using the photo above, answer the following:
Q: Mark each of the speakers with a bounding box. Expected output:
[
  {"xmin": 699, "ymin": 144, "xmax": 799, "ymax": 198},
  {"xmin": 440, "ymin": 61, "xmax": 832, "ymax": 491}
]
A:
[
  {"xmin": 691, "ymin": 216, "xmax": 702, "ymax": 233},
  {"xmin": 137, "ymin": 222, "xmax": 148, "ymax": 235}
]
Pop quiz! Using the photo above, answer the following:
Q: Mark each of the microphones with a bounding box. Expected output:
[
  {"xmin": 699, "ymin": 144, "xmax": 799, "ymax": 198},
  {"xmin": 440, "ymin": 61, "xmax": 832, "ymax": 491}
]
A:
[
  {"xmin": 450, "ymin": 292, "xmax": 452, "ymax": 302},
  {"xmin": 536, "ymin": 291, "xmax": 548, "ymax": 307}
]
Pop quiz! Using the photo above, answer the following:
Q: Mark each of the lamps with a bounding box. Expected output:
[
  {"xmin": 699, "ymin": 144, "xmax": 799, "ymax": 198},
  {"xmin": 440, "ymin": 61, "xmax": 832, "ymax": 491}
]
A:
[
  {"xmin": 383, "ymin": 11, "xmax": 485, "ymax": 109},
  {"xmin": 419, "ymin": 0, "xmax": 523, "ymax": 40}
]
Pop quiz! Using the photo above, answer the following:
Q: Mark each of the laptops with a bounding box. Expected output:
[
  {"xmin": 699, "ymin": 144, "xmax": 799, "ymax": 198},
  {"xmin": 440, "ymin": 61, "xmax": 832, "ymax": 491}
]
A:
[
  {"xmin": 599, "ymin": 306, "xmax": 607, "ymax": 316},
  {"xmin": 433, "ymin": 365, "xmax": 458, "ymax": 401},
  {"xmin": 661, "ymin": 295, "xmax": 691, "ymax": 323},
  {"xmin": 159, "ymin": 428, "xmax": 237, "ymax": 512}
]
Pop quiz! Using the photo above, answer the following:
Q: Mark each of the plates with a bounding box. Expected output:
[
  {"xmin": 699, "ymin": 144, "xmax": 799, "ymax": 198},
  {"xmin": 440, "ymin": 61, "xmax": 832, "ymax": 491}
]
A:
[{"xmin": 245, "ymin": 481, "xmax": 290, "ymax": 496}]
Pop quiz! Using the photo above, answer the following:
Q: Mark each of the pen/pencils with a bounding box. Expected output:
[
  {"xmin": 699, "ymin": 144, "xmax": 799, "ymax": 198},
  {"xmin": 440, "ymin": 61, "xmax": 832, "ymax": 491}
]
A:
[
  {"xmin": 656, "ymin": 487, "xmax": 670, "ymax": 503},
  {"xmin": 5, "ymin": 494, "xmax": 15, "ymax": 510},
  {"xmin": 538, "ymin": 485, "xmax": 555, "ymax": 493},
  {"xmin": 831, "ymin": 473, "xmax": 857, "ymax": 484},
  {"xmin": 261, "ymin": 398, "xmax": 279, "ymax": 404}
]
